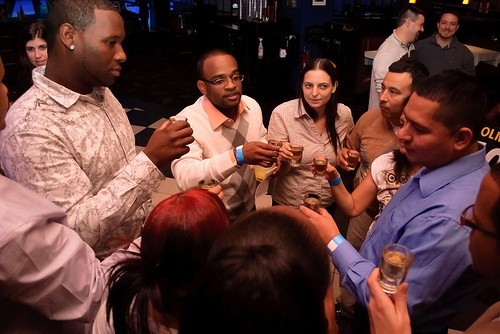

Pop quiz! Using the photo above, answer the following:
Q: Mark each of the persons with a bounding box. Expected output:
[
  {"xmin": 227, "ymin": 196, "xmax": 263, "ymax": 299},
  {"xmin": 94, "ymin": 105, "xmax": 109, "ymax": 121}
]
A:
[
  {"xmin": 0, "ymin": 57, "xmax": 103, "ymax": 334},
  {"xmin": 92, "ymin": 8, "xmax": 500, "ymax": 334},
  {"xmin": 0, "ymin": 0, "xmax": 194, "ymax": 262},
  {"xmin": 23, "ymin": 22, "xmax": 48, "ymax": 68}
]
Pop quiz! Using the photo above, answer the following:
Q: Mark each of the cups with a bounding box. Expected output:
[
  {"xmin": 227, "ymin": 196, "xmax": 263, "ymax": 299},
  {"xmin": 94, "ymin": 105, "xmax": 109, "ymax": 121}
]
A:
[
  {"xmin": 198, "ymin": 178, "xmax": 218, "ymax": 190},
  {"xmin": 169, "ymin": 115, "xmax": 187, "ymax": 125},
  {"xmin": 290, "ymin": 144, "xmax": 304, "ymax": 167},
  {"xmin": 377, "ymin": 244, "xmax": 413, "ymax": 295},
  {"xmin": 314, "ymin": 156, "xmax": 328, "ymax": 174},
  {"xmin": 268, "ymin": 139, "xmax": 283, "ymax": 158},
  {"xmin": 303, "ymin": 193, "xmax": 322, "ymax": 213}
]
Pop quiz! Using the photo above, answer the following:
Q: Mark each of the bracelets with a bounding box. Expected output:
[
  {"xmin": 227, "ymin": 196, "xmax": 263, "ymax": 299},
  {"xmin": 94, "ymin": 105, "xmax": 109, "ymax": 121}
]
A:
[
  {"xmin": 325, "ymin": 233, "xmax": 346, "ymax": 255},
  {"xmin": 329, "ymin": 177, "xmax": 342, "ymax": 186},
  {"xmin": 235, "ymin": 145, "xmax": 244, "ymax": 165}
]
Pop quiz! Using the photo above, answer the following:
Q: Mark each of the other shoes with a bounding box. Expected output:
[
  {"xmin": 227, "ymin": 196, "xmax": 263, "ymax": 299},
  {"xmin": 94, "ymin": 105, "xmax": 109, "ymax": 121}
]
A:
[{"xmin": 334, "ymin": 303, "xmax": 357, "ymax": 320}]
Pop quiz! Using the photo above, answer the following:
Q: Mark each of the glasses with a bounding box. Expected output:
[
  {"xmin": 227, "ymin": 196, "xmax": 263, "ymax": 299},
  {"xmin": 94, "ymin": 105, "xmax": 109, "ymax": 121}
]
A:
[
  {"xmin": 201, "ymin": 75, "xmax": 244, "ymax": 85},
  {"xmin": 460, "ymin": 205, "xmax": 497, "ymax": 238}
]
